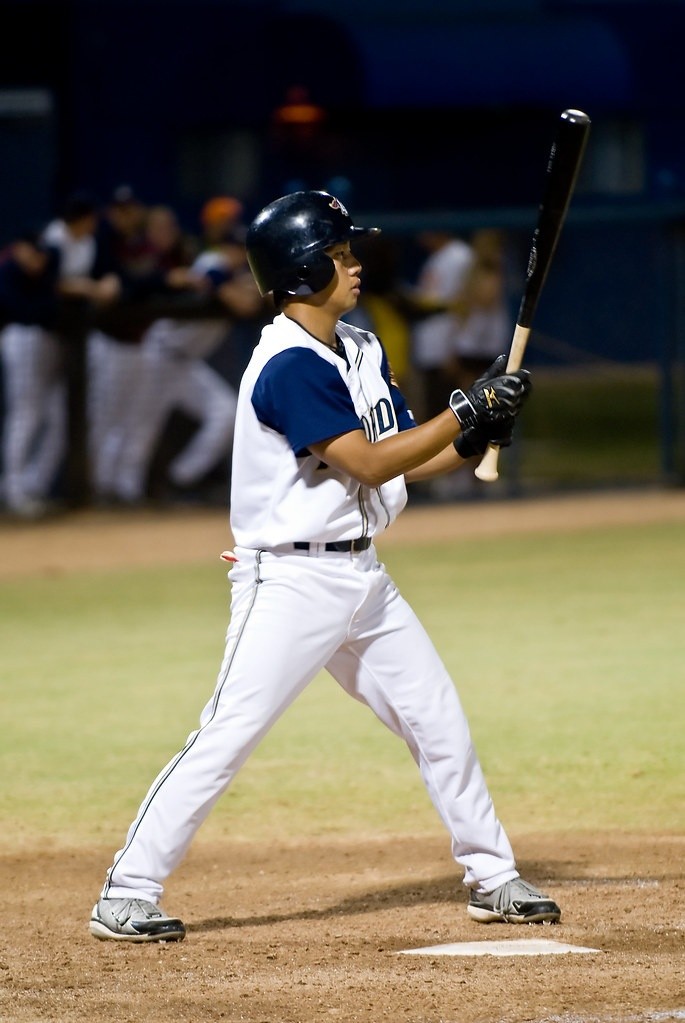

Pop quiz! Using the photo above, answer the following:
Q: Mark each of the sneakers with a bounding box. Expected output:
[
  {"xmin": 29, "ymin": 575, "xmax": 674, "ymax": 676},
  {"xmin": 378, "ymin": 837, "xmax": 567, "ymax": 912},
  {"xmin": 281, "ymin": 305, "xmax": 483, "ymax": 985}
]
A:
[
  {"xmin": 467, "ymin": 877, "xmax": 561, "ymax": 922},
  {"xmin": 89, "ymin": 899, "xmax": 186, "ymax": 941}
]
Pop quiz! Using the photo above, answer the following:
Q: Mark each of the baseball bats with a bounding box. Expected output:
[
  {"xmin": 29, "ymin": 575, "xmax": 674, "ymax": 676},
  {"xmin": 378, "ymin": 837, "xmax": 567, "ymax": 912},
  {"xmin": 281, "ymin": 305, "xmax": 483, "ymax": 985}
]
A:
[{"xmin": 473, "ymin": 109, "xmax": 593, "ymax": 484}]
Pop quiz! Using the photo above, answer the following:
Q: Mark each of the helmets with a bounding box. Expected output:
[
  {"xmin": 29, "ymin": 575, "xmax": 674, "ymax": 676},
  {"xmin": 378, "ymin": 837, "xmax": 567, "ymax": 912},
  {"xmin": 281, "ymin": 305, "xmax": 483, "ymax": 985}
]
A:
[{"xmin": 246, "ymin": 191, "xmax": 382, "ymax": 296}]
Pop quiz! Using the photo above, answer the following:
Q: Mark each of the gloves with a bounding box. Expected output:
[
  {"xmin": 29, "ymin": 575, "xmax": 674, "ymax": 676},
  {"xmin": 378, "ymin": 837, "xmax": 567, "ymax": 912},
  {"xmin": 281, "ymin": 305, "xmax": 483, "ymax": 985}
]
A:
[
  {"xmin": 452, "ymin": 421, "xmax": 513, "ymax": 459},
  {"xmin": 450, "ymin": 353, "xmax": 533, "ymax": 432}
]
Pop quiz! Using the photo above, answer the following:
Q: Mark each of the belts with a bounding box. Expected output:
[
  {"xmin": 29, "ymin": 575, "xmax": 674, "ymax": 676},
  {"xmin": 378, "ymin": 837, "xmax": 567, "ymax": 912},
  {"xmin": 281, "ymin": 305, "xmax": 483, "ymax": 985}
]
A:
[{"xmin": 293, "ymin": 538, "xmax": 372, "ymax": 552}]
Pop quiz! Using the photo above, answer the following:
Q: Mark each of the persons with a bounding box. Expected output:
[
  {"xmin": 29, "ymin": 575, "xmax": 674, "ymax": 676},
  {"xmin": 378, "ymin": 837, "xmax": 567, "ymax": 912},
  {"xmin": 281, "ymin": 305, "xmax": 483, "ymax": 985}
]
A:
[
  {"xmin": 89, "ymin": 189, "xmax": 562, "ymax": 942},
  {"xmin": 0, "ymin": 195, "xmax": 516, "ymax": 521}
]
